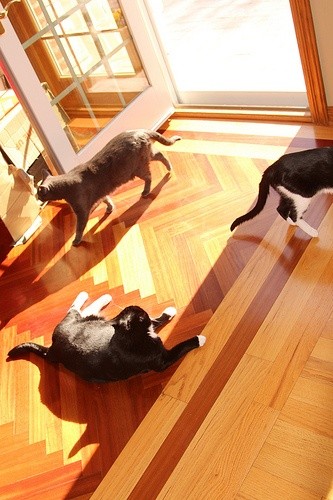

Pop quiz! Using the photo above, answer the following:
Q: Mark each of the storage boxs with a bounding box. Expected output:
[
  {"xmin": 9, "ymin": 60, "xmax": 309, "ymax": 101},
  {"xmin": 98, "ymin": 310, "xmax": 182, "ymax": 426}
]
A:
[{"xmin": 0, "ymin": 82, "xmax": 80, "ymax": 242}]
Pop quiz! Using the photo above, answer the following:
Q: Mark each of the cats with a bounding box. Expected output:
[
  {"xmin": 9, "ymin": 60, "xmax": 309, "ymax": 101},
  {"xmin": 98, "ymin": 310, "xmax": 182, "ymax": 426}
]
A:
[
  {"xmin": 230, "ymin": 147, "xmax": 333, "ymax": 237},
  {"xmin": 35, "ymin": 129, "xmax": 181, "ymax": 246},
  {"xmin": 7, "ymin": 292, "xmax": 206, "ymax": 384}
]
